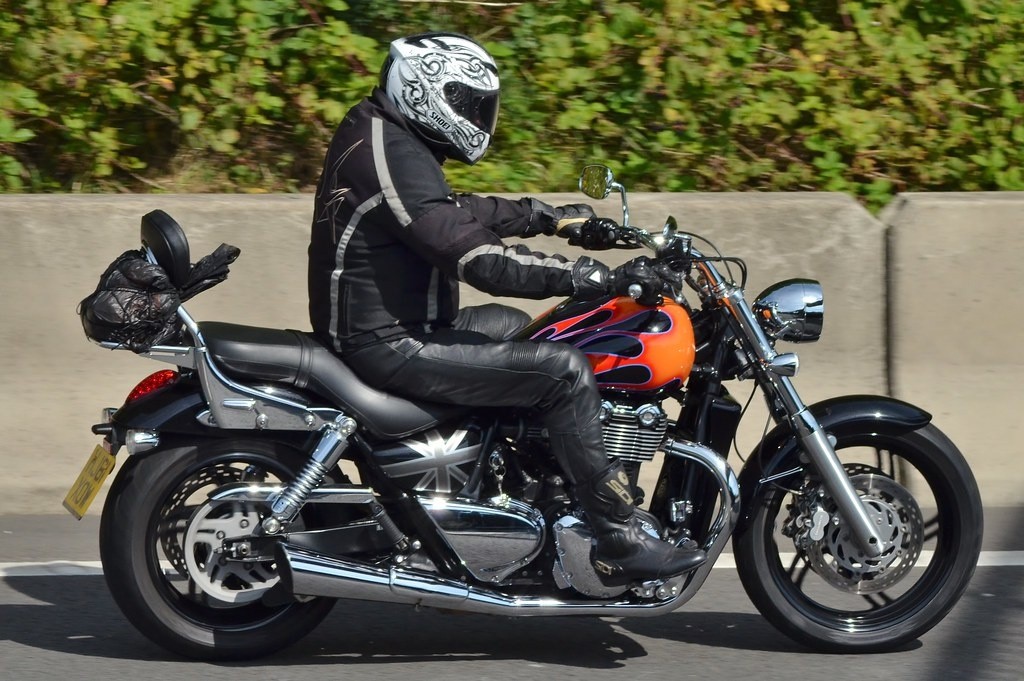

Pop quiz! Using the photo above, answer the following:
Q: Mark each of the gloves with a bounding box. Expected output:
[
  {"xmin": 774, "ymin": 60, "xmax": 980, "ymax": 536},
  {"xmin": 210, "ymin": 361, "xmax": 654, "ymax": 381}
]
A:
[
  {"xmin": 571, "ymin": 254, "xmax": 682, "ymax": 302},
  {"xmin": 521, "ymin": 196, "xmax": 597, "ymax": 238}
]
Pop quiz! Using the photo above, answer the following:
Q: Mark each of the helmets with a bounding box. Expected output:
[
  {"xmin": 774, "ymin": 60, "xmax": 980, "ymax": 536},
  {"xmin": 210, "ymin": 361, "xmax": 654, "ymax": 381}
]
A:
[{"xmin": 379, "ymin": 29, "xmax": 501, "ymax": 167}]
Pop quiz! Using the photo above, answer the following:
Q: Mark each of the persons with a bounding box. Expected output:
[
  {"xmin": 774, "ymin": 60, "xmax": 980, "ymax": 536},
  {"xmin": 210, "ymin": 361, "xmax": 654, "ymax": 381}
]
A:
[{"xmin": 307, "ymin": 26, "xmax": 713, "ymax": 589}]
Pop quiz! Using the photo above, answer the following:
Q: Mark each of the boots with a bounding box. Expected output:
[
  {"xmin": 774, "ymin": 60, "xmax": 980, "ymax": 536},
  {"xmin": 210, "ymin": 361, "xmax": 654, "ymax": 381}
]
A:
[{"xmin": 572, "ymin": 456, "xmax": 707, "ymax": 588}]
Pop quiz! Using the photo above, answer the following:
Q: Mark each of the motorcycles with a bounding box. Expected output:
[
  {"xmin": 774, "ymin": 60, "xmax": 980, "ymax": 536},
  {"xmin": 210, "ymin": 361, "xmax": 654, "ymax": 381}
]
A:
[{"xmin": 62, "ymin": 163, "xmax": 986, "ymax": 653}]
